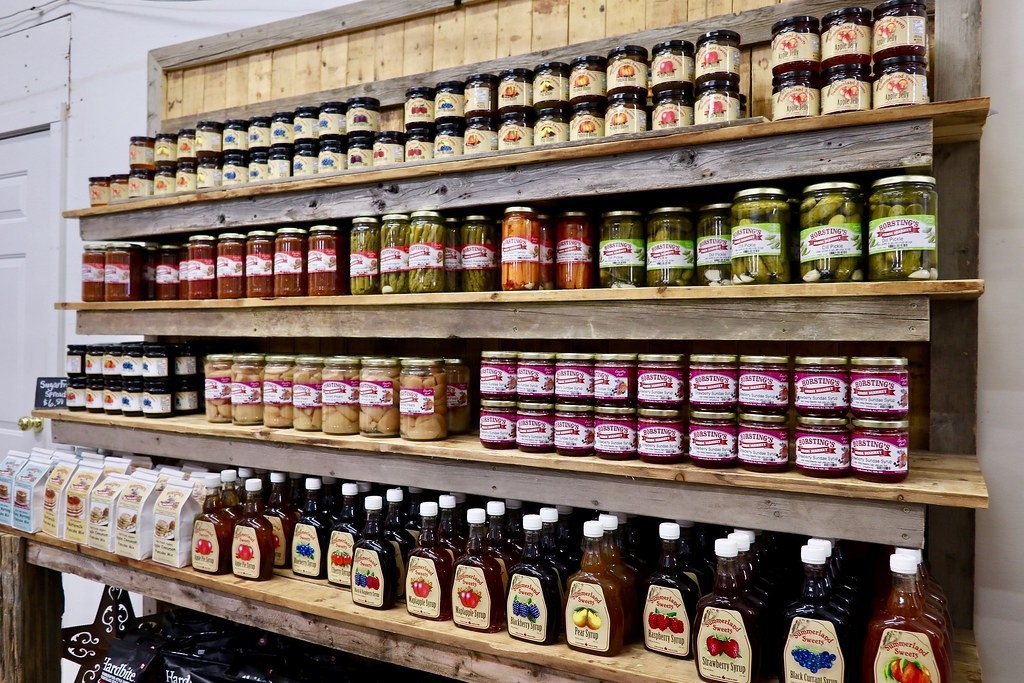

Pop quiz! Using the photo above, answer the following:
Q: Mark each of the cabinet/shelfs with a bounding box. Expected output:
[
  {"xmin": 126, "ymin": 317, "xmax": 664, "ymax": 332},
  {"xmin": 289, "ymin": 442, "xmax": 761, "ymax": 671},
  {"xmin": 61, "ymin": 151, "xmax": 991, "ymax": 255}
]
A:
[{"xmin": 0, "ymin": 0, "xmax": 986, "ymax": 682}]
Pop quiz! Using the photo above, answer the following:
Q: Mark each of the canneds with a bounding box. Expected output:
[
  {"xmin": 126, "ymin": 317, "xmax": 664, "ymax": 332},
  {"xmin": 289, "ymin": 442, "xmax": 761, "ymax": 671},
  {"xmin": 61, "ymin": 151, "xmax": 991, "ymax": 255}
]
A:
[{"xmin": 62, "ymin": 0, "xmax": 940, "ymax": 482}]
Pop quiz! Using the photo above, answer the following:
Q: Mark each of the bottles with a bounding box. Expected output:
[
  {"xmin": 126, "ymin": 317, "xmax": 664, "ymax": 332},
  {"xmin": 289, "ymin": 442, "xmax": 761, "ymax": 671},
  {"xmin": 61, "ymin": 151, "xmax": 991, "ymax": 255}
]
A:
[{"xmin": 195, "ymin": 467, "xmax": 961, "ymax": 683}]
